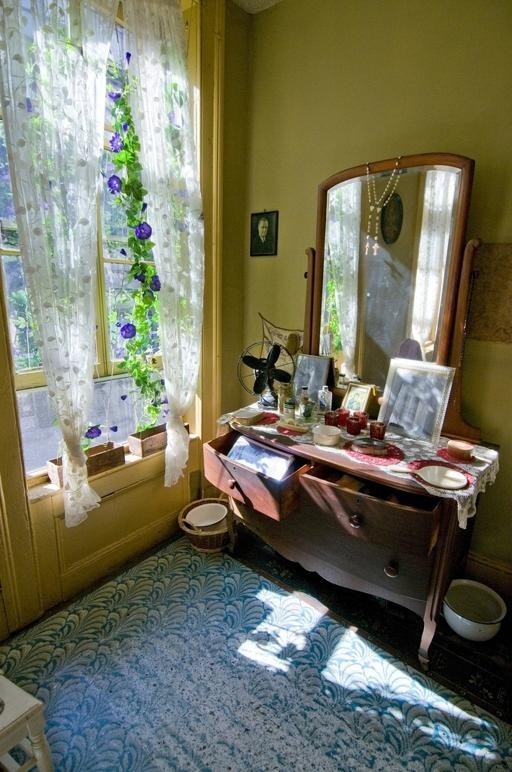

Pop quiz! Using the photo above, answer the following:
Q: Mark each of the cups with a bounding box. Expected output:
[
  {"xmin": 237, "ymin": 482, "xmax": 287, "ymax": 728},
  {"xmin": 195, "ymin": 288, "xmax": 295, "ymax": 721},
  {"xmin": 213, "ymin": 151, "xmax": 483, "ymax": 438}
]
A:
[{"xmin": 324, "ymin": 408, "xmax": 386, "ymax": 440}]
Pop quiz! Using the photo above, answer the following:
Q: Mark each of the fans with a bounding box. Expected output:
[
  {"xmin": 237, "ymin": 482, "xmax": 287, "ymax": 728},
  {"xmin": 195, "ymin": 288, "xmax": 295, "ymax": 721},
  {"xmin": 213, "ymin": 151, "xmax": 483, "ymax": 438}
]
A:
[{"xmin": 237, "ymin": 341, "xmax": 294, "ymax": 407}]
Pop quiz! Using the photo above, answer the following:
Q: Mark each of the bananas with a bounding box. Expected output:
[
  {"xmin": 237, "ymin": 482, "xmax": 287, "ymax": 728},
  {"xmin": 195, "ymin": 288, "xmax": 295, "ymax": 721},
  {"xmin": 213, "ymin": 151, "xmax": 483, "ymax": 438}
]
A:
[{"xmin": 229, "ymin": 412, "xmax": 266, "ymax": 426}]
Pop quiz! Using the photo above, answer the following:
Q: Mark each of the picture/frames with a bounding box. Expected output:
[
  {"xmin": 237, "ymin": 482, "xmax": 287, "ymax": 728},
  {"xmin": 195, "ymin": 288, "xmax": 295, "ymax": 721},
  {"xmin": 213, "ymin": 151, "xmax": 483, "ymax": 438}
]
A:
[
  {"xmin": 376, "ymin": 358, "xmax": 456, "ymax": 447},
  {"xmin": 381, "ymin": 193, "xmax": 404, "ymax": 243},
  {"xmin": 294, "ymin": 353, "xmax": 332, "ymax": 402},
  {"xmin": 250, "ymin": 211, "xmax": 278, "ymax": 256},
  {"xmin": 338, "ymin": 383, "xmax": 372, "ymax": 420}
]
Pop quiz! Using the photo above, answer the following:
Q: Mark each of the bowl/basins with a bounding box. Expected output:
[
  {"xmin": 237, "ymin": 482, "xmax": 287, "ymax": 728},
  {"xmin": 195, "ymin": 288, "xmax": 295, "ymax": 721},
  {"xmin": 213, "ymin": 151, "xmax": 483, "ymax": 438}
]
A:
[
  {"xmin": 313, "ymin": 425, "xmax": 342, "ymax": 446},
  {"xmin": 442, "ymin": 577, "xmax": 507, "ymax": 642},
  {"xmin": 184, "ymin": 502, "xmax": 228, "ymax": 532}
]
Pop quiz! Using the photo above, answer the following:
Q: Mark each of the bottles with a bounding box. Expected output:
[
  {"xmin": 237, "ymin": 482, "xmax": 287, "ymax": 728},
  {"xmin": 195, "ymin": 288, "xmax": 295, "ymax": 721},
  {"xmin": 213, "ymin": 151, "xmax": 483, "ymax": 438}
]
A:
[{"xmin": 277, "ymin": 383, "xmax": 332, "ymax": 421}]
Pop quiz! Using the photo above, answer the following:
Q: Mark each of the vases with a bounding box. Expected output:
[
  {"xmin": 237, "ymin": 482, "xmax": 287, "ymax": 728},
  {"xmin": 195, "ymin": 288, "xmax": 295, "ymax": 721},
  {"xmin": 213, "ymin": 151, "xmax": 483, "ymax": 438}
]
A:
[
  {"xmin": 45, "ymin": 442, "xmax": 125, "ymax": 486},
  {"xmin": 129, "ymin": 423, "xmax": 192, "ymax": 458}
]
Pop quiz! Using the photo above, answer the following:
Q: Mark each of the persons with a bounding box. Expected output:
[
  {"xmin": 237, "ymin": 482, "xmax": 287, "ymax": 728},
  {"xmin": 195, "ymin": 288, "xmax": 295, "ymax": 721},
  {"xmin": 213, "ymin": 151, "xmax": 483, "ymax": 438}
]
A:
[
  {"xmin": 389, "ymin": 373, "xmax": 438, "ymax": 438},
  {"xmin": 347, "ymin": 393, "xmax": 361, "ymax": 410},
  {"xmin": 252, "ymin": 217, "xmax": 273, "ymax": 253}
]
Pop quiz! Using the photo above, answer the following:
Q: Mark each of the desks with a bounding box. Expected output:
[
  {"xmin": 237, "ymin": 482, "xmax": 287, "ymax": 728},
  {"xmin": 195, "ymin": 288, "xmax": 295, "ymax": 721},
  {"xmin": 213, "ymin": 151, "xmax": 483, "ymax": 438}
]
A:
[
  {"xmin": 202, "ymin": 399, "xmax": 501, "ymax": 673},
  {"xmin": 0, "ymin": 673, "xmax": 56, "ymax": 772}
]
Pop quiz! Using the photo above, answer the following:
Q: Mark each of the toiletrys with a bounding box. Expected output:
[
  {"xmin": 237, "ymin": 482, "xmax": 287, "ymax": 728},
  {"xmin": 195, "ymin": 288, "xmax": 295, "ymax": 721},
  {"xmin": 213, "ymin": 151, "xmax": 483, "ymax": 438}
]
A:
[
  {"xmin": 317, "ymin": 386, "xmax": 332, "ymax": 414},
  {"xmin": 283, "ymin": 381, "xmax": 299, "ymax": 414},
  {"xmin": 277, "ymin": 382, "xmax": 290, "ymax": 414},
  {"xmin": 299, "ymin": 385, "xmax": 316, "ymax": 422}
]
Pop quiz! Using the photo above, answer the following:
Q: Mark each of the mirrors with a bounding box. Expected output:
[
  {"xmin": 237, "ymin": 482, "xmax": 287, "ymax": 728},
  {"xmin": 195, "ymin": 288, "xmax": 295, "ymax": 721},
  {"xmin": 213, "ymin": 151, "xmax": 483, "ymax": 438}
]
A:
[{"xmin": 301, "ymin": 153, "xmax": 481, "ymax": 444}]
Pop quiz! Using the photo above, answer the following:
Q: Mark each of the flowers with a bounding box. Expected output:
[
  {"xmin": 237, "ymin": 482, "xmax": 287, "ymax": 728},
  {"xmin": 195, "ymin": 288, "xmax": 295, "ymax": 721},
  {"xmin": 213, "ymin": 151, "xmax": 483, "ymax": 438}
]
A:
[
  {"xmin": 109, "ymin": 88, "xmax": 168, "ymax": 428},
  {"xmin": 80, "ymin": 424, "xmax": 117, "ymax": 450}
]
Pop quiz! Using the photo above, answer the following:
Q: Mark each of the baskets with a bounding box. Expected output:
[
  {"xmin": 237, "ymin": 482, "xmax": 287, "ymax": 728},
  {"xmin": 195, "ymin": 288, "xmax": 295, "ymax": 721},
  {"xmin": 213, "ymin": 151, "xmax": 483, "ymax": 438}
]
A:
[{"xmin": 178, "ymin": 492, "xmax": 232, "ymax": 554}]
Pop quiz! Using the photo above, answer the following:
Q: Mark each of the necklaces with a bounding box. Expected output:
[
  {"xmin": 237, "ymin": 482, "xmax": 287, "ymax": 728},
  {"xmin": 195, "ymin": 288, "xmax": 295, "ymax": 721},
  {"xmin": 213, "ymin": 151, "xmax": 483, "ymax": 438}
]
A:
[
  {"xmin": 372, "ymin": 170, "xmax": 404, "ymax": 266},
  {"xmin": 364, "ymin": 155, "xmax": 400, "ymax": 257}
]
